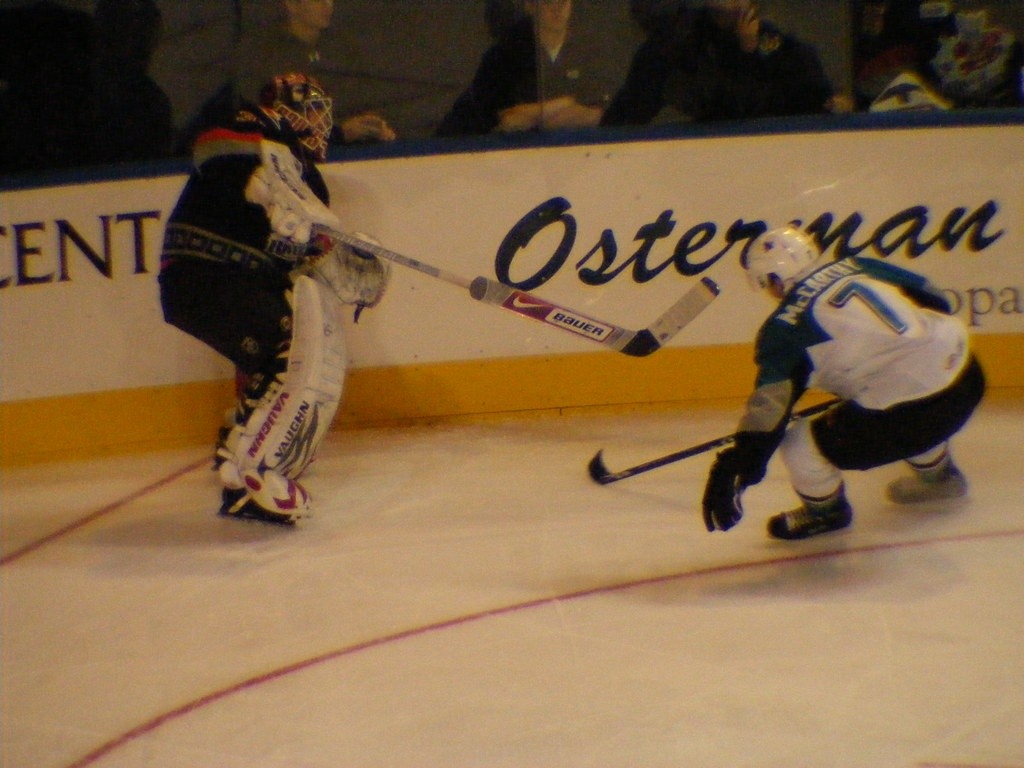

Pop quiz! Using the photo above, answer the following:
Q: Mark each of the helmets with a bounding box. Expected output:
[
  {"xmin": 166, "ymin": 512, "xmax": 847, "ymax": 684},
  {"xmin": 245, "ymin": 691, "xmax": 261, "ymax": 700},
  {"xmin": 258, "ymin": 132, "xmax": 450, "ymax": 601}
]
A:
[
  {"xmin": 259, "ymin": 72, "xmax": 334, "ymax": 163},
  {"xmin": 745, "ymin": 228, "xmax": 822, "ymax": 294}
]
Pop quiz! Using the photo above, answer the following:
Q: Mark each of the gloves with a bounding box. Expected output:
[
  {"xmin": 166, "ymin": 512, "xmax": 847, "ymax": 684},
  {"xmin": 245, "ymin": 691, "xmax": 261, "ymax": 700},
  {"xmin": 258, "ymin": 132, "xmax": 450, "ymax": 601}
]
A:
[{"xmin": 703, "ymin": 452, "xmax": 755, "ymax": 532}]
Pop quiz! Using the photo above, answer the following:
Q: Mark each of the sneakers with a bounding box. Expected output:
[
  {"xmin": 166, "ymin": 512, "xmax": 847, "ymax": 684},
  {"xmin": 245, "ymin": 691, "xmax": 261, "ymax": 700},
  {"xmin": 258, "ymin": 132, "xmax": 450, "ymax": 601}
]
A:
[
  {"xmin": 213, "ymin": 429, "xmax": 231, "ymax": 468},
  {"xmin": 220, "ymin": 487, "xmax": 291, "ymax": 522},
  {"xmin": 766, "ymin": 500, "xmax": 854, "ymax": 553},
  {"xmin": 887, "ymin": 459, "xmax": 968, "ymax": 508}
]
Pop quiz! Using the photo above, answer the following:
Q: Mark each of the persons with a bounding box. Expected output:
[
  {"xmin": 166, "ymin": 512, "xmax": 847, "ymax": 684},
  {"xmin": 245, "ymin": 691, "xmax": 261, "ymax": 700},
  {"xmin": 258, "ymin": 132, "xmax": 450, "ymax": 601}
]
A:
[
  {"xmin": 701, "ymin": 226, "xmax": 988, "ymax": 540},
  {"xmin": 598, "ymin": 0, "xmax": 833, "ymax": 129},
  {"xmin": 432, "ymin": 0, "xmax": 601, "ymax": 139},
  {"xmin": 228, "ymin": 0, "xmax": 396, "ymax": 142},
  {"xmin": 158, "ymin": 74, "xmax": 349, "ymax": 517}
]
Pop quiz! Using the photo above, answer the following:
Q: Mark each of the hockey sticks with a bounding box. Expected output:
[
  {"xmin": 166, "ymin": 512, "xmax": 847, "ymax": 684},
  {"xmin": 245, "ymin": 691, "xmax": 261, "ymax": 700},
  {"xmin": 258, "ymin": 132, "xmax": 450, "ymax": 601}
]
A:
[
  {"xmin": 588, "ymin": 396, "xmax": 844, "ymax": 487},
  {"xmin": 313, "ymin": 221, "xmax": 723, "ymax": 360}
]
